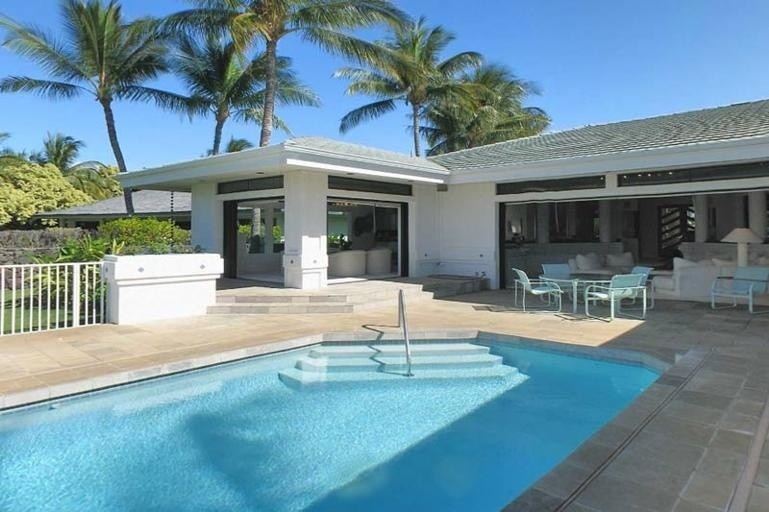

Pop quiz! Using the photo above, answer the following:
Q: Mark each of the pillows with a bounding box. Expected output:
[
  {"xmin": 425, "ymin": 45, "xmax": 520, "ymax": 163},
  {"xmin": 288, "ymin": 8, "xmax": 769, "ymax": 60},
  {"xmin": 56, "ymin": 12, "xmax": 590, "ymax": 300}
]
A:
[
  {"xmin": 570, "ymin": 251, "xmax": 635, "ymax": 271},
  {"xmin": 673, "ymin": 257, "xmax": 736, "ymax": 279}
]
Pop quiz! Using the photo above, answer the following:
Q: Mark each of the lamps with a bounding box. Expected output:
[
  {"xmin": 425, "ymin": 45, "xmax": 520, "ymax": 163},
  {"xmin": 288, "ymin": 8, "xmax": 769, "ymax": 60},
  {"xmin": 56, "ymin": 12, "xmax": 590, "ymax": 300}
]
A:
[{"xmin": 721, "ymin": 228, "xmax": 764, "ymax": 267}]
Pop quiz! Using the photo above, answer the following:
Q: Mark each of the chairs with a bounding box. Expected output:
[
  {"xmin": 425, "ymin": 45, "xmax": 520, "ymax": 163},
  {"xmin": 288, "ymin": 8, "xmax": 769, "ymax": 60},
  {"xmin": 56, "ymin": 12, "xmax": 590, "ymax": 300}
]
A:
[
  {"xmin": 710, "ymin": 266, "xmax": 769, "ymax": 316},
  {"xmin": 510, "ymin": 263, "xmax": 655, "ymax": 322}
]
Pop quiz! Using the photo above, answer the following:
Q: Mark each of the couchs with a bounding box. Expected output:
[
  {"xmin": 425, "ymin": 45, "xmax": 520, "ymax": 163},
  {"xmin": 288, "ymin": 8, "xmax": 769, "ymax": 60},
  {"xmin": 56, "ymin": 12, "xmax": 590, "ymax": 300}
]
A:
[{"xmin": 650, "ymin": 265, "xmax": 734, "ymax": 301}]
